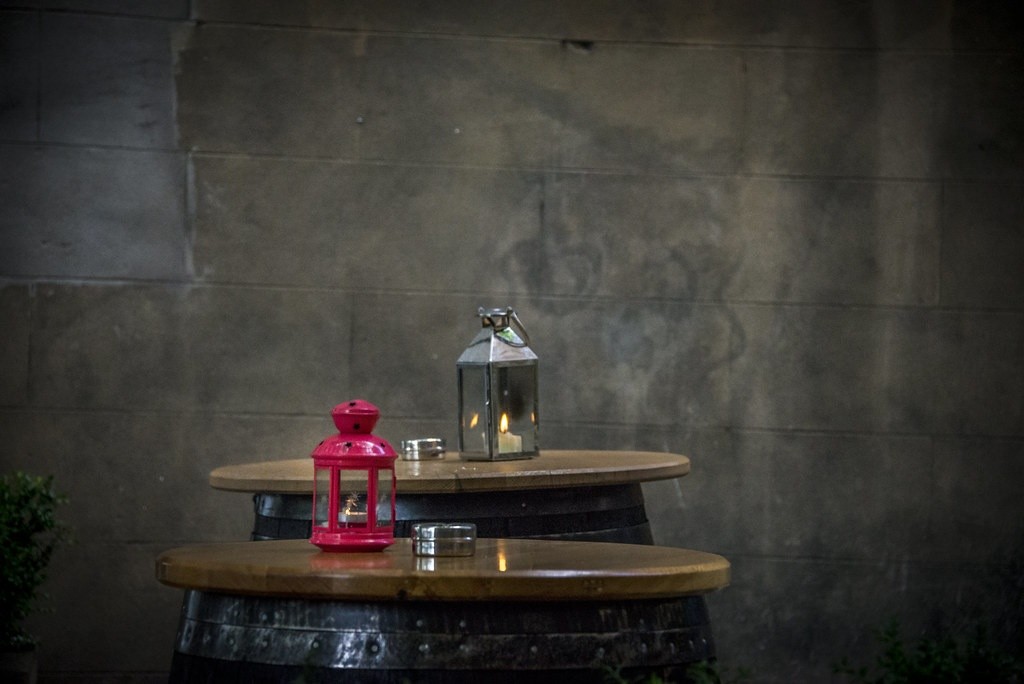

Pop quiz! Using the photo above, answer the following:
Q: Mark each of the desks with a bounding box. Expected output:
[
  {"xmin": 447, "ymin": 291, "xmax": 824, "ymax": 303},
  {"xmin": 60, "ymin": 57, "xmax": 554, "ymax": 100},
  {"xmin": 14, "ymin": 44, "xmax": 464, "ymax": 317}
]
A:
[
  {"xmin": 209, "ymin": 450, "xmax": 690, "ymax": 544},
  {"xmin": 153, "ymin": 537, "xmax": 730, "ymax": 684}
]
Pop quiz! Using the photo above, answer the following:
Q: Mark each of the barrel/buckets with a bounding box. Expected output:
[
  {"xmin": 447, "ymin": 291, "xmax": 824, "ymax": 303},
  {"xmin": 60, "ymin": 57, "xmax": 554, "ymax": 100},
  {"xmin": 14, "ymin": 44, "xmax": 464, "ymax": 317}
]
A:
[
  {"xmin": 208, "ymin": 448, "xmax": 691, "ymax": 545},
  {"xmin": 154, "ymin": 535, "xmax": 731, "ymax": 684}
]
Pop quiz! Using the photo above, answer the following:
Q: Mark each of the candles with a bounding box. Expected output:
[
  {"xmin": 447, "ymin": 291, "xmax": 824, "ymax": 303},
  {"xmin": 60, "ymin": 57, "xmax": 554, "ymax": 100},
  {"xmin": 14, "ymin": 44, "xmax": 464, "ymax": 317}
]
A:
[{"xmin": 483, "ymin": 414, "xmax": 522, "ymax": 453}]
